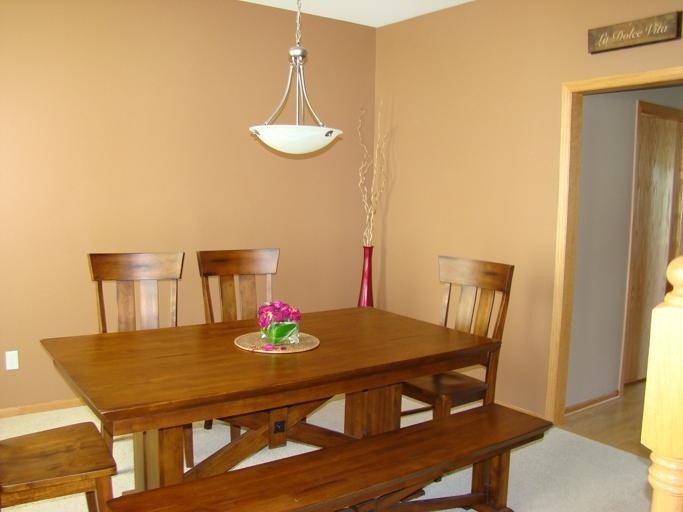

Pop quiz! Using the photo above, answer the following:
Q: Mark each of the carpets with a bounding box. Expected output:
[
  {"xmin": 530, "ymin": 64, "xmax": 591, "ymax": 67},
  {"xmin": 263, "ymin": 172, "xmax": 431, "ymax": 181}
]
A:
[{"xmin": 0, "ymin": 394, "xmax": 652, "ymax": 512}]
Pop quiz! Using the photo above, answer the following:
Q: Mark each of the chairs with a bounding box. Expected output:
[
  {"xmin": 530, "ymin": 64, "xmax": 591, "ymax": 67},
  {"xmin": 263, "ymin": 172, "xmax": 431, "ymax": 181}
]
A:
[
  {"xmin": 87, "ymin": 252, "xmax": 193, "ymax": 468},
  {"xmin": 401, "ymin": 256, "xmax": 514, "ymax": 482},
  {"xmin": 0, "ymin": 421, "xmax": 117, "ymax": 512},
  {"xmin": 196, "ymin": 247, "xmax": 318, "ymax": 442}
]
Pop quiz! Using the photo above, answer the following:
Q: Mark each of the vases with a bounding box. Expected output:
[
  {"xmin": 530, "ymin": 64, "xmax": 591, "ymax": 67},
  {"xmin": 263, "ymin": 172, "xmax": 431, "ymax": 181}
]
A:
[{"xmin": 358, "ymin": 246, "xmax": 373, "ymax": 308}]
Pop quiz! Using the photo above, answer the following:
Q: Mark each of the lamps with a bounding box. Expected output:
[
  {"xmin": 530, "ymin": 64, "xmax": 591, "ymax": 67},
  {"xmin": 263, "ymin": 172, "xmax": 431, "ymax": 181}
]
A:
[{"xmin": 249, "ymin": 0, "xmax": 343, "ymax": 155}]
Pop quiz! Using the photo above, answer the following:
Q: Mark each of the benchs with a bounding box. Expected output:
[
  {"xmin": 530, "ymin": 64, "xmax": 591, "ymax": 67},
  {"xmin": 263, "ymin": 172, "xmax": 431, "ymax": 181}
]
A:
[{"xmin": 105, "ymin": 402, "xmax": 553, "ymax": 512}]
